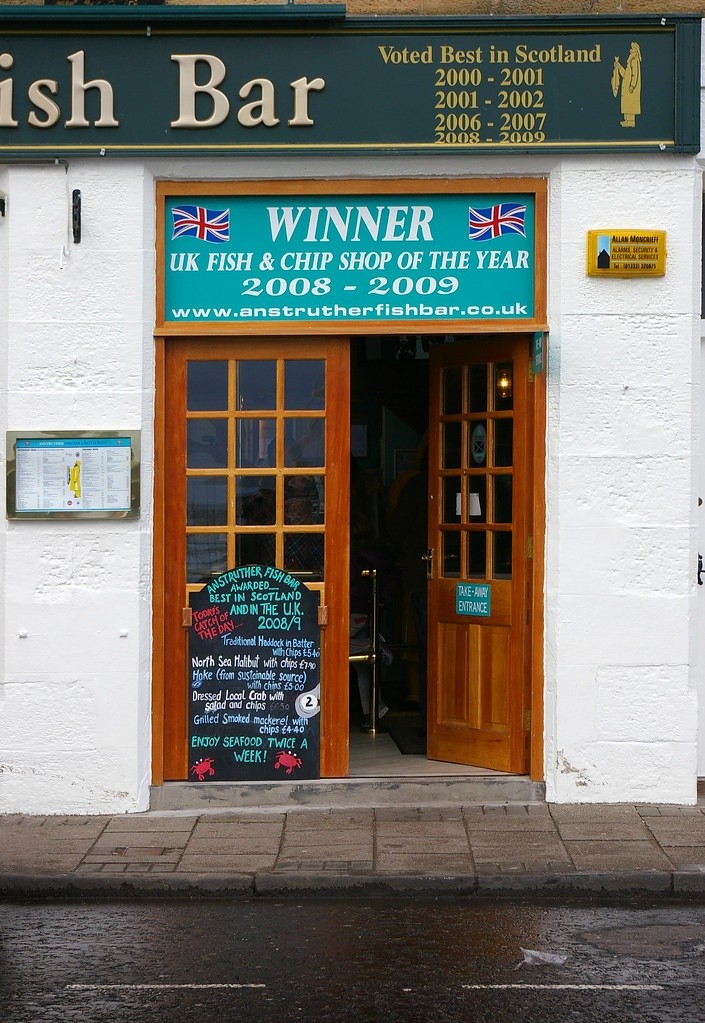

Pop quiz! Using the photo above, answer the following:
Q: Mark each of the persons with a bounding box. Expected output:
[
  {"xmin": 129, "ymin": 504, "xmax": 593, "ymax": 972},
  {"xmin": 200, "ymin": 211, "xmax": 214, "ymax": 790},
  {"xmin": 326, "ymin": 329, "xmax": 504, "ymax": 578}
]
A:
[
  {"xmin": 387, "ymin": 450, "xmax": 429, "ymax": 740},
  {"xmin": 185, "ymin": 419, "xmax": 227, "ymax": 584},
  {"xmin": 244, "ymin": 436, "xmax": 324, "ymax": 579}
]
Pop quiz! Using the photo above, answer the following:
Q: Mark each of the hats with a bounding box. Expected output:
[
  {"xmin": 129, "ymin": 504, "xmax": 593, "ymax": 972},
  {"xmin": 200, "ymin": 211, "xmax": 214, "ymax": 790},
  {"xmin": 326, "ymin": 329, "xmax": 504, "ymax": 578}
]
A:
[{"xmin": 188, "ymin": 419, "xmax": 217, "ymax": 444}]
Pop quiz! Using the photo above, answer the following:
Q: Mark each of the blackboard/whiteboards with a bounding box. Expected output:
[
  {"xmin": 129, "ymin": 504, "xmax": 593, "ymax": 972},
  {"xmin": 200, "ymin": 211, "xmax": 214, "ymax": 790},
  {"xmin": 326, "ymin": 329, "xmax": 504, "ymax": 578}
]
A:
[{"xmin": 186, "ymin": 564, "xmax": 321, "ymax": 783}]
